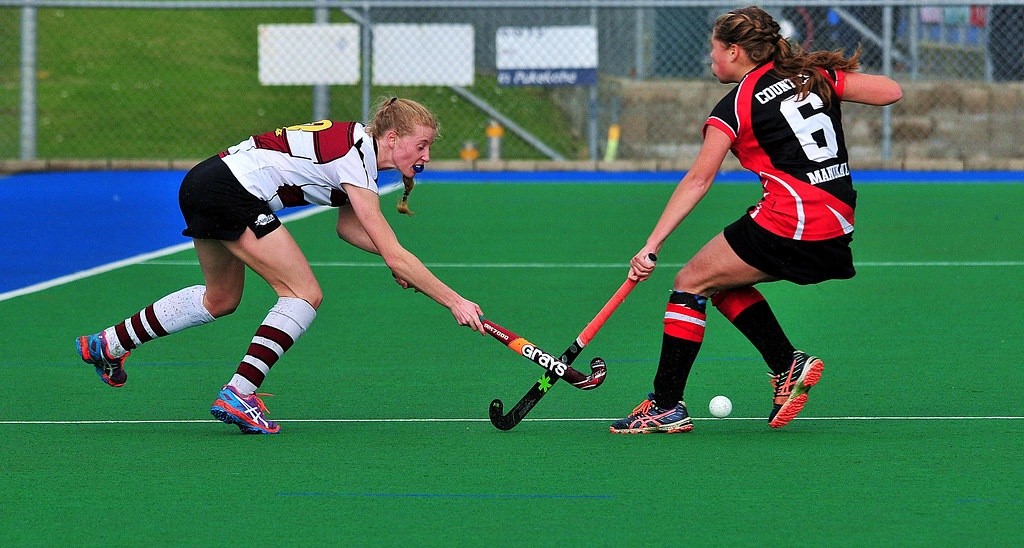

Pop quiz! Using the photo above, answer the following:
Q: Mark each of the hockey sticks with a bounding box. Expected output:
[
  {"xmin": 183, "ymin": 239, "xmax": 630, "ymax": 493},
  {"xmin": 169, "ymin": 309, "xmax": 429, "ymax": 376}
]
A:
[
  {"xmin": 408, "ymin": 277, "xmax": 608, "ymax": 391},
  {"xmin": 488, "ymin": 252, "xmax": 658, "ymax": 430}
]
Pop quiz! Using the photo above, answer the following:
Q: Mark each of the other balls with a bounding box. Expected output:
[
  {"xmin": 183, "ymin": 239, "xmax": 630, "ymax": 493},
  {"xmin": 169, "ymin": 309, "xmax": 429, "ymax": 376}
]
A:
[{"xmin": 707, "ymin": 395, "xmax": 733, "ymax": 418}]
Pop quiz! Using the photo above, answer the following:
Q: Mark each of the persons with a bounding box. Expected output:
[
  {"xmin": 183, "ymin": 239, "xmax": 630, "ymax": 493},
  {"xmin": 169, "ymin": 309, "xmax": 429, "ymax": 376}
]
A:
[
  {"xmin": 609, "ymin": 6, "xmax": 903, "ymax": 434},
  {"xmin": 76, "ymin": 95, "xmax": 487, "ymax": 435}
]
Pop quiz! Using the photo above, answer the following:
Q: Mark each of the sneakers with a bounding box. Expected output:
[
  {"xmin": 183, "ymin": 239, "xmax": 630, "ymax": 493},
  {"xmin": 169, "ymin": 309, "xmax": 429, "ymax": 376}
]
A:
[
  {"xmin": 210, "ymin": 384, "xmax": 280, "ymax": 433},
  {"xmin": 75, "ymin": 329, "xmax": 131, "ymax": 387},
  {"xmin": 610, "ymin": 393, "xmax": 694, "ymax": 433},
  {"xmin": 765, "ymin": 350, "xmax": 825, "ymax": 429}
]
